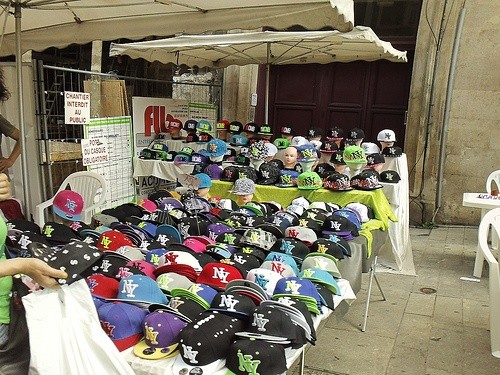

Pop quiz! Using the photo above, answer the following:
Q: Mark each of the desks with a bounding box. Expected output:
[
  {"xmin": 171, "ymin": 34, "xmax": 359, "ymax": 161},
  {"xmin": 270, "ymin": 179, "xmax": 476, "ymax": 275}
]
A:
[
  {"xmin": 120, "ymin": 137, "xmax": 414, "ymax": 375},
  {"xmin": 462, "ymin": 192, "xmax": 500, "ymax": 276}
]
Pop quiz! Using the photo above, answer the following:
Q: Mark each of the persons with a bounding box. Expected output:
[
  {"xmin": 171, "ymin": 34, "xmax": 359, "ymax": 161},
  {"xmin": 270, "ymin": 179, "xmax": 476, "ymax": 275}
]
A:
[
  {"xmin": 0, "ymin": 173, "xmax": 68, "ymax": 350},
  {"xmin": 0, "ymin": 65, "xmax": 27, "ymax": 182},
  {"xmin": 192, "ymin": 173, "xmax": 212, "ymax": 201},
  {"xmin": 168, "ymin": 119, "xmax": 367, "ymax": 176},
  {"xmin": 229, "ymin": 177, "xmax": 255, "ymax": 204},
  {"xmin": 377, "ymin": 129, "xmax": 396, "ymax": 151}
]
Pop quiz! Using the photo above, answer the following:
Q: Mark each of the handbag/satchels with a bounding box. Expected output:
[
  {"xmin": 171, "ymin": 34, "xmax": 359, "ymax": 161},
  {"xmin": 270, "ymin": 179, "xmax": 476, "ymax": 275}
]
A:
[
  {"xmin": 20, "ymin": 279, "xmax": 135, "ymax": 375},
  {"xmin": 0, "ymin": 276, "xmax": 33, "ymax": 375}
]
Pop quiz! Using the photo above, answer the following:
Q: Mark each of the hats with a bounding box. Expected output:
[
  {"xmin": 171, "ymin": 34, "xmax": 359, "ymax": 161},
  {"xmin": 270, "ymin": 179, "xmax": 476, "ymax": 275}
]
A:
[
  {"xmin": 183, "ymin": 120, "xmax": 198, "ymax": 131},
  {"xmin": 280, "ymin": 126, "xmax": 296, "ymax": 134},
  {"xmin": 258, "ymin": 124, "xmax": 273, "ymax": 135},
  {"xmin": 228, "ymin": 121, "xmax": 243, "ymax": 134},
  {"xmin": 243, "ymin": 123, "xmax": 260, "ymax": 134},
  {"xmin": 326, "ymin": 128, "xmax": 344, "ymax": 140},
  {"xmin": 138, "ymin": 136, "xmax": 401, "ymax": 196},
  {"xmin": 345, "ymin": 128, "xmax": 365, "ymax": 141},
  {"xmin": 377, "ymin": 129, "xmax": 396, "ymax": 142},
  {"xmin": 167, "ymin": 119, "xmax": 183, "ymax": 130},
  {"xmin": 216, "ymin": 120, "xmax": 229, "ymax": 130},
  {"xmin": 196, "ymin": 120, "xmax": 211, "ymax": 133},
  {"xmin": 6, "ymin": 189, "xmax": 376, "ymax": 375},
  {"xmin": 307, "ymin": 127, "xmax": 323, "ymax": 137}
]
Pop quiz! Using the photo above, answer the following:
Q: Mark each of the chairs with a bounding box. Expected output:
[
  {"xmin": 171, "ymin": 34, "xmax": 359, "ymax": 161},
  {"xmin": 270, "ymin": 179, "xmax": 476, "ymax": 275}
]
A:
[
  {"xmin": 37, "ymin": 170, "xmax": 106, "ymax": 231},
  {"xmin": 478, "ymin": 169, "xmax": 500, "ymax": 359}
]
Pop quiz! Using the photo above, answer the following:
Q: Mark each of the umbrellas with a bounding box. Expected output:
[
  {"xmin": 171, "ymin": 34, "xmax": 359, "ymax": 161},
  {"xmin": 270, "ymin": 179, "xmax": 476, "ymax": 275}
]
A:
[
  {"xmin": 110, "ymin": 26, "xmax": 409, "ymax": 116},
  {"xmin": 0, "ymin": 0, "xmax": 355, "ymax": 207}
]
